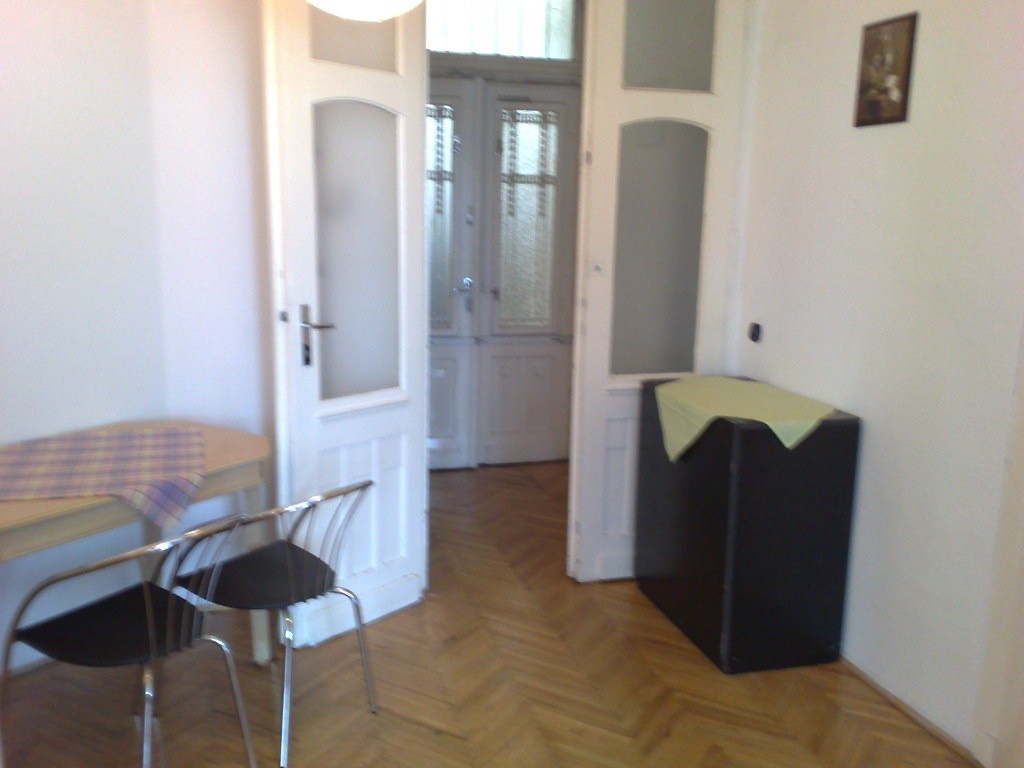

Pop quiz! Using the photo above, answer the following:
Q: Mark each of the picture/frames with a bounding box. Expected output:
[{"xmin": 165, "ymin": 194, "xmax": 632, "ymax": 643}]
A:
[{"xmin": 854, "ymin": 13, "xmax": 916, "ymax": 127}]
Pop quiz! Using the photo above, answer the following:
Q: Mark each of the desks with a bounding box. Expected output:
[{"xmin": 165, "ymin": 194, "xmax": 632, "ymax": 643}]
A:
[{"xmin": 0, "ymin": 419, "xmax": 273, "ymax": 666}]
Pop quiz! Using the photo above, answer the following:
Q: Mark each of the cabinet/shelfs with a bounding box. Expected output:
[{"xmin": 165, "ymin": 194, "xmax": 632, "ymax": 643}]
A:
[{"xmin": 632, "ymin": 375, "xmax": 860, "ymax": 674}]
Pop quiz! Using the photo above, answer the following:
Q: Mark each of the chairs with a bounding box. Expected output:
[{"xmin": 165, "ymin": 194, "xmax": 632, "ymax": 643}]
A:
[{"xmin": 0, "ymin": 479, "xmax": 380, "ymax": 768}]
